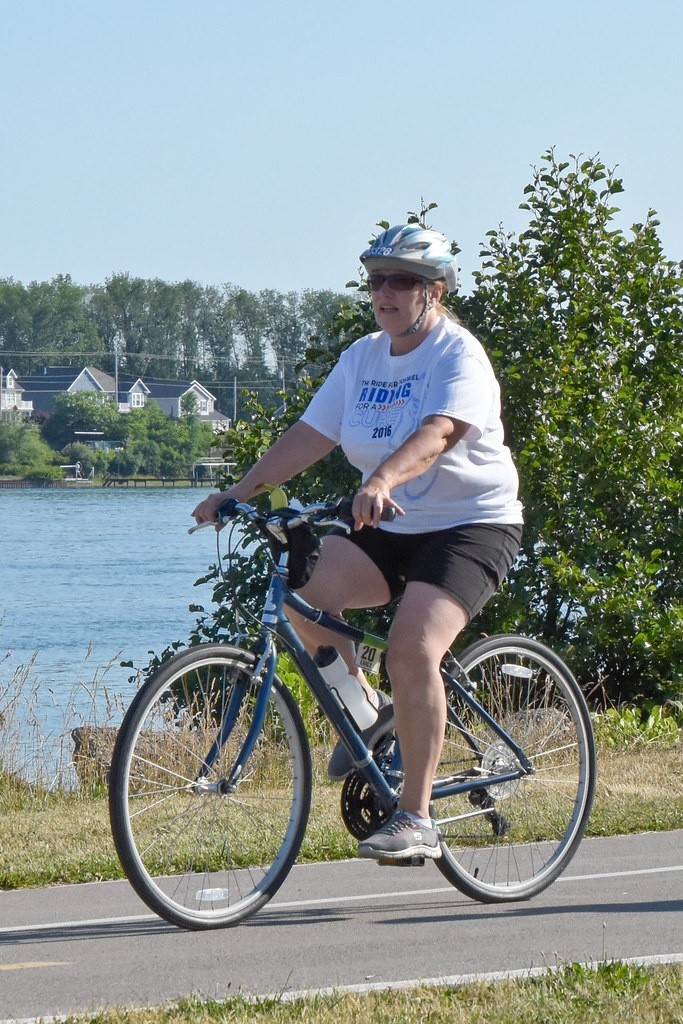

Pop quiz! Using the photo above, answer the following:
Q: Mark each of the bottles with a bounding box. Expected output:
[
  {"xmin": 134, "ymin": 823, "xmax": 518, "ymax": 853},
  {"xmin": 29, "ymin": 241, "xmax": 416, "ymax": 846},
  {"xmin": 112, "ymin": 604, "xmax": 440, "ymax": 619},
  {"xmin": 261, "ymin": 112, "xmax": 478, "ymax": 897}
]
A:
[{"xmin": 312, "ymin": 644, "xmax": 379, "ymax": 731}]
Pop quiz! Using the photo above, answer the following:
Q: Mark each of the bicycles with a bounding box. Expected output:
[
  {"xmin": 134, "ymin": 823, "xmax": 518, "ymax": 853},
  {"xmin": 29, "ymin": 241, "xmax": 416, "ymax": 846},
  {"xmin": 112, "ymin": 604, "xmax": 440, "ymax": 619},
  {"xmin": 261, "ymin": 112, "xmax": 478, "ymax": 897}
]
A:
[{"xmin": 108, "ymin": 497, "xmax": 598, "ymax": 932}]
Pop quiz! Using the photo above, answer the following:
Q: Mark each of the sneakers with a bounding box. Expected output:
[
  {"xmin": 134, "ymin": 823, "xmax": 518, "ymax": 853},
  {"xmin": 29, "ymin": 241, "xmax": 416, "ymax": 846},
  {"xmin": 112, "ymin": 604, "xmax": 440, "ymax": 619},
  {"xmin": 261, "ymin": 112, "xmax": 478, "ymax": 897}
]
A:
[
  {"xmin": 358, "ymin": 811, "xmax": 442, "ymax": 860},
  {"xmin": 327, "ymin": 687, "xmax": 394, "ymax": 781}
]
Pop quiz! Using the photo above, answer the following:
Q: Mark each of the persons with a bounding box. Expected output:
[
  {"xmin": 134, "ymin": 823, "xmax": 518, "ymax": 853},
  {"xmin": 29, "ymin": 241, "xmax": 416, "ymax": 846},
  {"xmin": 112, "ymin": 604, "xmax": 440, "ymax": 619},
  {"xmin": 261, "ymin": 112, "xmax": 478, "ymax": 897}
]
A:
[{"xmin": 193, "ymin": 223, "xmax": 524, "ymax": 860}]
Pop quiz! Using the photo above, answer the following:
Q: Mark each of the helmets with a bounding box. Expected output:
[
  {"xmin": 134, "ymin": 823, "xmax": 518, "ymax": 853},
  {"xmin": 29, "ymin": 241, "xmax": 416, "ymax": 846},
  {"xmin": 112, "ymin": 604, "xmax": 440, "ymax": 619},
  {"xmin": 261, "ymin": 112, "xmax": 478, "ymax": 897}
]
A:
[{"xmin": 359, "ymin": 223, "xmax": 457, "ymax": 292}]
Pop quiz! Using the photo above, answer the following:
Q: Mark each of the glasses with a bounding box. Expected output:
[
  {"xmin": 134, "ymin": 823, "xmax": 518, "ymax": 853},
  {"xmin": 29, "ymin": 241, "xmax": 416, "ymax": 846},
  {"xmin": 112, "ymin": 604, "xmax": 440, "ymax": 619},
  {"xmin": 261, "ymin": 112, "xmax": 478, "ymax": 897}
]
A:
[{"xmin": 367, "ymin": 273, "xmax": 439, "ymax": 290}]
